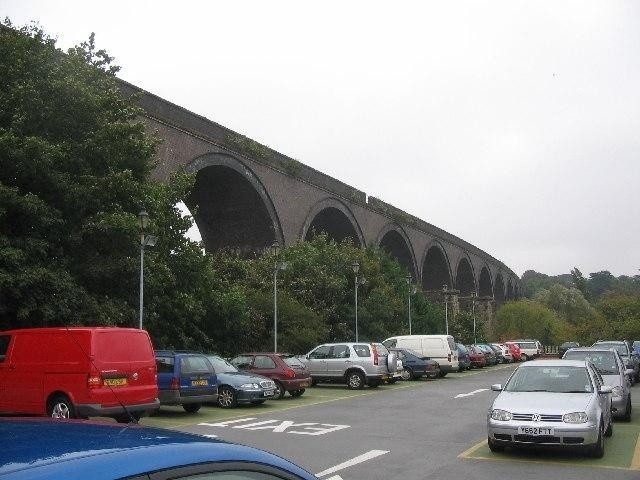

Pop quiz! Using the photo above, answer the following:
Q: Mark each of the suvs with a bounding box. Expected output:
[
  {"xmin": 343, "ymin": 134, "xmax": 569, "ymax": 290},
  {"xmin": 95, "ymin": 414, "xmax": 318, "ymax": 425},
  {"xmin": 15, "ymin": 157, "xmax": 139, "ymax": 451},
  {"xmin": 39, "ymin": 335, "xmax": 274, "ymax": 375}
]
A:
[{"xmin": 297, "ymin": 343, "xmax": 396, "ymax": 390}]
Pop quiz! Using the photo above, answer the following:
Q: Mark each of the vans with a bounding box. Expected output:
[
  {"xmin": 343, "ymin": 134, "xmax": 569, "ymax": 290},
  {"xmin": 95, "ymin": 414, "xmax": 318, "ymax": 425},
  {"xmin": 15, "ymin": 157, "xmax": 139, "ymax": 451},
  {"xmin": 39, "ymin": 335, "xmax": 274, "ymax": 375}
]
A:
[
  {"xmin": 382, "ymin": 335, "xmax": 459, "ymax": 377},
  {"xmin": 513, "ymin": 341, "xmax": 543, "ymax": 362},
  {"xmin": 0, "ymin": 327, "xmax": 161, "ymax": 425},
  {"xmin": 150, "ymin": 349, "xmax": 219, "ymax": 417}
]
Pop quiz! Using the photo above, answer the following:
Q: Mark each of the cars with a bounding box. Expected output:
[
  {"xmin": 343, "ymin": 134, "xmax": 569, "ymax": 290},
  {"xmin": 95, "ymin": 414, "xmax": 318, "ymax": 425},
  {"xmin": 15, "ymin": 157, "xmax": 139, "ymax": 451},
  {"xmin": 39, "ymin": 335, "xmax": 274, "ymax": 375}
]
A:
[
  {"xmin": 558, "ymin": 342, "xmax": 580, "ymax": 359},
  {"xmin": 203, "ymin": 355, "xmax": 278, "ymax": 409},
  {"xmin": 486, "ymin": 360, "xmax": 612, "ymax": 458},
  {"xmin": 0, "ymin": 420, "xmax": 320, "ymax": 479},
  {"xmin": 388, "ymin": 348, "xmax": 441, "ymax": 381},
  {"xmin": 228, "ymin": 352, "xmax": 312, "ymax": 400},
  {"xmin": 590, "ymin": 339, "xmax": 639, "ymax": 386},
  {"xmin": 455, "ymin": 342, "xmax": 521, "ymax": 369},
  {"xmin": 561, "ymin": 347, "xmax": 635, "ymax": 422}
]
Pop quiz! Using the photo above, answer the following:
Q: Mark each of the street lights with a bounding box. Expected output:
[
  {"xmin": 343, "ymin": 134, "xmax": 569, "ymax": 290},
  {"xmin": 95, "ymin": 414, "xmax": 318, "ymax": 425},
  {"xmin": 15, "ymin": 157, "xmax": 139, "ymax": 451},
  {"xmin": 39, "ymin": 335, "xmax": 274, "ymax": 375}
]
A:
[
  {"xmin": 405, "ymin": 273, "xmax": 417, "ymax": 335},
  {"xmin": 138, "ymin": 200, "xmax": 158, "ymax": 329},
  {"xmin": 271, "ymin": 240, "xmax": 289, "ymax": 352},
  {"xmin": 352, "ymin": 258, "xmax": 366, "ymax": 343},
  {"xmin": 471, "ymin": 291, "xmax": 477, "ymax": 343},
  {"xmin": 443, "ymin": 283, "xmax": 449, "ymax": 334}
]
